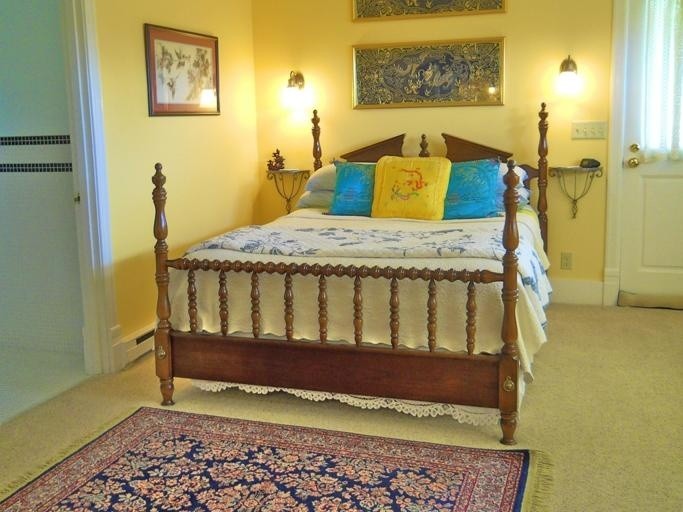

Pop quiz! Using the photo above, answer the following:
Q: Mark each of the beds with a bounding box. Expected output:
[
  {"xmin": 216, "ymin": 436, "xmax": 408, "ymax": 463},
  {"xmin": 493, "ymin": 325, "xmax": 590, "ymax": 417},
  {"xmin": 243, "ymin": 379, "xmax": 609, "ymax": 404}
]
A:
[{"xmin": 152, "ymin": 101, "xmax": 548, "ymax": 446}]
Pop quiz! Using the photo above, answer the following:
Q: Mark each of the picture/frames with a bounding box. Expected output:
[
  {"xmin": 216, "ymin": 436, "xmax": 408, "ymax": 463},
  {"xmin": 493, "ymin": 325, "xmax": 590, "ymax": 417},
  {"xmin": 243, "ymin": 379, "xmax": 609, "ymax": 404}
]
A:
[
  {"xmin": 350, "ymin": 0, "xmax": 506, "ymax": 21},
  {"xmin": 142, "ymin": 22, "xmax": 223, "ymax": 117},
  {"xmin": 349, "ymin": 34, "xmax": 504, "ymax": 109}
]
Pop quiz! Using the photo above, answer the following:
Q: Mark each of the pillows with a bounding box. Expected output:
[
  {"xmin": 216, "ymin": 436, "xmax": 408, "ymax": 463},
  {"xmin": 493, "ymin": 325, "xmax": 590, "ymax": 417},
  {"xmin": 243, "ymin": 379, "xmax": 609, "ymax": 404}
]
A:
[{"xmin": 297, "ymin": 156, "xmax": 535, "ymax": 223}]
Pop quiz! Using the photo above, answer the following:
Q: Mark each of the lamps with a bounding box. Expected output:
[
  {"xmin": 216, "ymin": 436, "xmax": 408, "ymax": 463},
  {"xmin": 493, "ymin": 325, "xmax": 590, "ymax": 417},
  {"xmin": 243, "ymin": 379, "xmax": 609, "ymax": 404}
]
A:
[
  {"xmin": 280, "ymin": 70, "xmax": 303, "ymax": 111},
  {"xmin": 553, "ymin": 51, "xmax": 581, "ymax": 95}
]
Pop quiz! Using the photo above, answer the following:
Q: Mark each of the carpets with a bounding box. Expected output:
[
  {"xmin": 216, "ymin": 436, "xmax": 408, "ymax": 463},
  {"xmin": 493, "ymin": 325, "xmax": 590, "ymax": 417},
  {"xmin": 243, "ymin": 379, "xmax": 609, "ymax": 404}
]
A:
[{"xmin": 0, "ymin": 406, "xmax": 549, "ymax": 511}]
